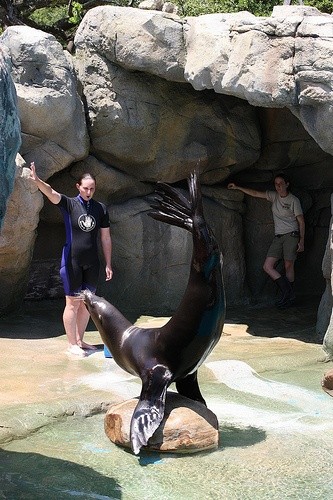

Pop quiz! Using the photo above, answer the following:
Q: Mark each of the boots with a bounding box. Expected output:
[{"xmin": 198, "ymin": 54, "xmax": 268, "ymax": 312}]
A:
[
  {"xmin": 279, "ymin": 282, "xmax": 296, "ymax": 309},
  {"xmin": 272, "ymin": 276, "xmax": 287, "ymax": 307}
]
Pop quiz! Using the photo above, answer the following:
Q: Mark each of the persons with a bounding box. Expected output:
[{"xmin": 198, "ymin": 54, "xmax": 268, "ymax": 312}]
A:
[
  {"xmin": 227, "ymin": 172, "xmax": 305, "ymax": 310},
  {"xmin": 26, "ymin": 162, "xmax": 113, "ymax": 355}
]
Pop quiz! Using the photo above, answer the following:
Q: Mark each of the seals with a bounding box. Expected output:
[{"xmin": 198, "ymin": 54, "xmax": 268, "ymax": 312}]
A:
[{"xmin": 72, "ymin": 158, "xmax": 226, "ymax": 455}]
